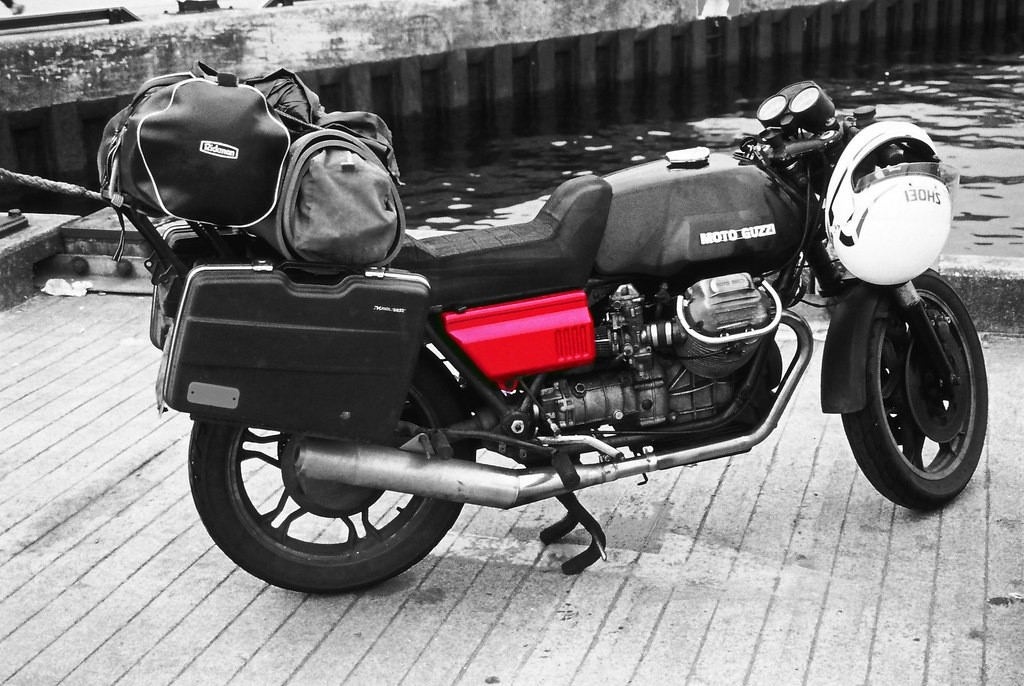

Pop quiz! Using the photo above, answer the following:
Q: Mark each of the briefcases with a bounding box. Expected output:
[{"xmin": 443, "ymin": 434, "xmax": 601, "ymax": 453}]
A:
[{"xmin": 156, "ymin": 260, "xmax": 430, "ymax": 438}]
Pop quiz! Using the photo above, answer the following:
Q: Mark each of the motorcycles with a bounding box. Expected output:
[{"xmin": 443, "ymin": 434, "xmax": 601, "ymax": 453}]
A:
[{"xmin": 150, "ymin": 78, "xmax": 990, "ymax": 597}]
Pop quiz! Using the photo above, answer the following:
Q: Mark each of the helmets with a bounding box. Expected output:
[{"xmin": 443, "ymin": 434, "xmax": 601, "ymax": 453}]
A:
[{"xmin": 823, "ymin": 120, "xmax": 952, "ymax": 285}]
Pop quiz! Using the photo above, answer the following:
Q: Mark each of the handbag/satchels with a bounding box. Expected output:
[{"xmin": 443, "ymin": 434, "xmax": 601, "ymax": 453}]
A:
[{"xmin": 97, "ymin": 72, "xmax": 293, "ymax": 229}]
